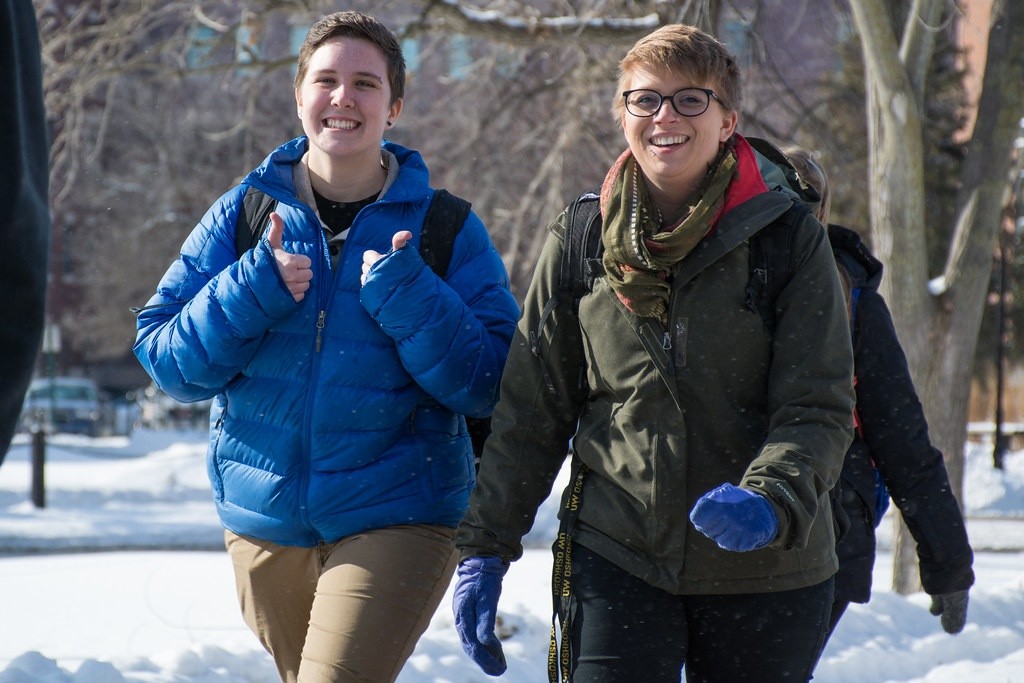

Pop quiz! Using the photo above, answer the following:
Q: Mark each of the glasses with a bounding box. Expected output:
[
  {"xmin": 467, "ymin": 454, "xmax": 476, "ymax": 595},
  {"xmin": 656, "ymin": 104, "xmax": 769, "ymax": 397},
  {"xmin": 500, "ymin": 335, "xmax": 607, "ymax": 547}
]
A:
[{"xmin": 623, "ymin": 87, "xmax": 729, "ymax": 117}]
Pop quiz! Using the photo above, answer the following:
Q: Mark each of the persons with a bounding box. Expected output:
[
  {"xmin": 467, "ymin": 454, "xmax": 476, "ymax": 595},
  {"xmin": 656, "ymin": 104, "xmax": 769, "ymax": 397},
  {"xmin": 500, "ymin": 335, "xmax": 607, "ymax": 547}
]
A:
[
  {"xmin": 452, "ymin": 23, "xmax": 855, "ymax": 682},
  {"xmin": 132, "ymin": 14, "xmax": 521, "ymax": 683},
  {"xmin": 781, "ymin": 146, "xmax": 976, "ymax": 637}
]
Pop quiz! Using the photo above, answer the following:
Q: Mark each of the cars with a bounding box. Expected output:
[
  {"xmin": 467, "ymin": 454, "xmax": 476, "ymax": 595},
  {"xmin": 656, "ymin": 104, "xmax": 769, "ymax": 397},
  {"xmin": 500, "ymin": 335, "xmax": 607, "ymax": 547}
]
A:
[{"xmin": 21, "ymin": 377, "xmax": 99, "ymax": 435}]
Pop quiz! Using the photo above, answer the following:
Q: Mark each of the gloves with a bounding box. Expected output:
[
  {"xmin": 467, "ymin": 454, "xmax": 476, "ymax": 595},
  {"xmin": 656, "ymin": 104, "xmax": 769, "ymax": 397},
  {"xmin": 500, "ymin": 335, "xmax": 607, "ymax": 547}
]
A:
[
  {"xmin": 452, "ymin": 556, "xmax": 507, "ymax": 676},
  {"xmin": 689, "ymin": 483, "xmax": 779, "ymax": 552},
  {"xmin": 930, "ymin": 589, "xmax": 968, "ymax": 634}
]
